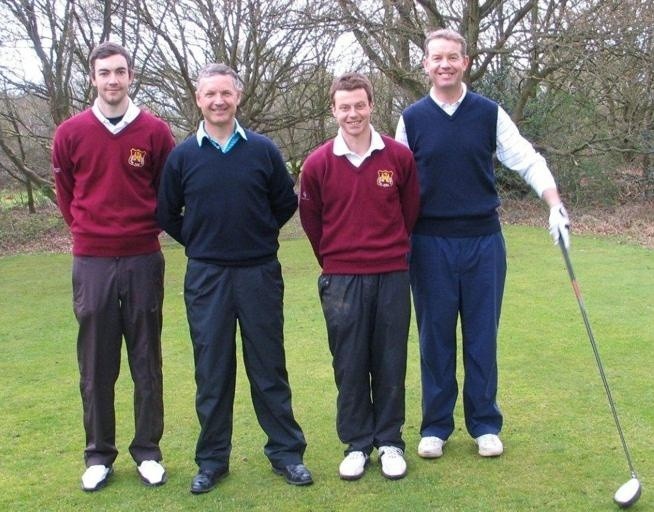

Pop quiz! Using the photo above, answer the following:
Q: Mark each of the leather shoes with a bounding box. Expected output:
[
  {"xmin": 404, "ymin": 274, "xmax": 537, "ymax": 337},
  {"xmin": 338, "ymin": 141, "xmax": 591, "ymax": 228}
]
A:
[
  {"xmin": 192, "ymin": 466, "xmax": 228, "ymax": 492},
  {"xmin": 271, "ymin": 462, "xmax": 312, "ymax": 486}
]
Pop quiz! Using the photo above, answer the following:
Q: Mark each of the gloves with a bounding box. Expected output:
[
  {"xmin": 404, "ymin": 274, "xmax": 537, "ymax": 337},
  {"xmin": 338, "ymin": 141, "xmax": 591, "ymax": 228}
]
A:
[{"xmin": 549, "ymin": 204, "xmax": 571, "ymax": 250}]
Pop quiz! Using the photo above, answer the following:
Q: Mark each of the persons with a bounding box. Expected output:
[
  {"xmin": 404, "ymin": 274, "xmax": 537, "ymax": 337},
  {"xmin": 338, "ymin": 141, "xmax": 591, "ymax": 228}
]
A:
[
  {"xmin": 298, "ymin": 73, "xmax": 419, "ymax": 481},
  {"xmin": 53, "ymin": 42, "xmax": 177, "ymax": 490},
  {"xmin": 156, "ymin": 65, "xmax": 313, "ymax": 494},
  {"xmin": 395, "ymin": 31, "xmax": 572, "ymax": 459}
]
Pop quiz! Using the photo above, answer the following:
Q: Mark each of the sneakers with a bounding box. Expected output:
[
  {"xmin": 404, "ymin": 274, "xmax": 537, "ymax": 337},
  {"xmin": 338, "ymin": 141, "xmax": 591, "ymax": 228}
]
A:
[
  {"xmin": 476, "ymin": 433, "xmax": 504, "ymax": 455},
  {"xmin": 377, "ymin": 445, "xmax": 410, "ymax": 479},
  {"xmin": 81, "ymin": 463, "xmax": 113, "ymax": 491},
  {"xmin": 417, "ymin": 435, "xmax": 445, "ymax": 457},
  {"xmin": 339, "ymin": 450, "xmax": 370, "ymax": 480},
  {"xmin": 136, "ymin": 461, "xmax": 166, "ymax": 485}
]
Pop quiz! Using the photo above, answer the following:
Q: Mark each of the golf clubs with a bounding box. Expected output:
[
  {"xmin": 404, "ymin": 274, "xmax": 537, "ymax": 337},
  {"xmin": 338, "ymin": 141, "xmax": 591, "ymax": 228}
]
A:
[{"xmin": 557, "ymin": 231, "xmax": 642, "ymax": 506}]
